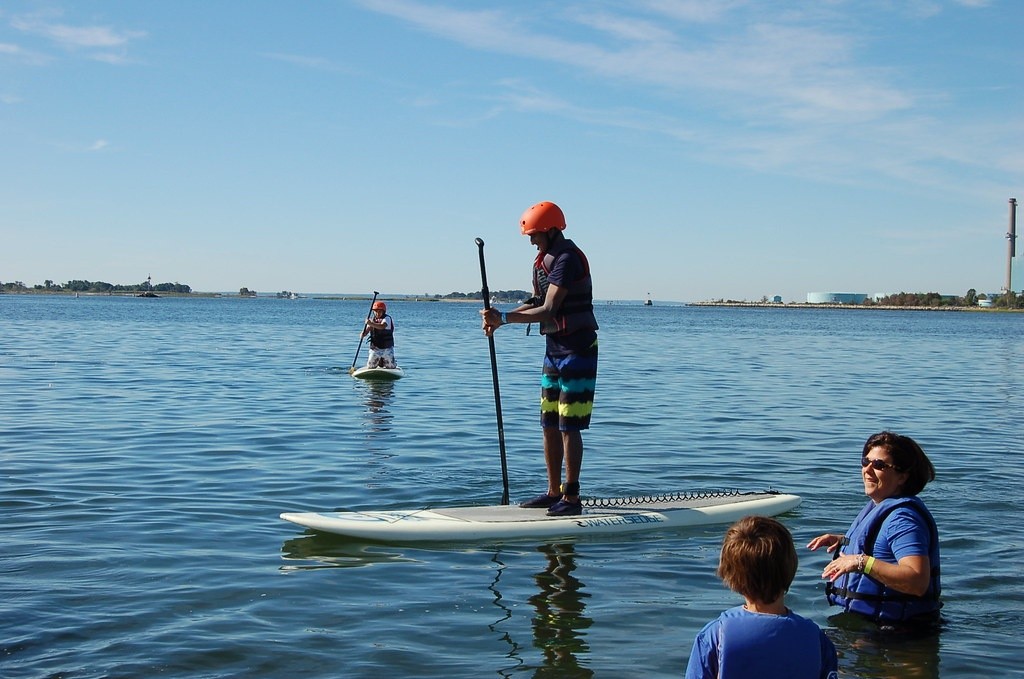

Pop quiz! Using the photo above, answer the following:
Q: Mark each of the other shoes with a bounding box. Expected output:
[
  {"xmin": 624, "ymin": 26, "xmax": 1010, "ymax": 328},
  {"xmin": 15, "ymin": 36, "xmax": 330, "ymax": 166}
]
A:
[
  {"xmin": 545, "ymin": 499, "xmax": 582, "ymax": 515},
  {"xmin": 521, "ymin": 493, "xmax": 560, "ymax": 507}
]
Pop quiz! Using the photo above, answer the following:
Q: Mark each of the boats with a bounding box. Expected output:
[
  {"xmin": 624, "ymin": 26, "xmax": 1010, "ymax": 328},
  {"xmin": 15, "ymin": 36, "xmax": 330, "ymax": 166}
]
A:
[
  {"xmin": 489, "ymin": 295, "xmax": 500, "ymax": 303},
  {"xmin": 644, "ymin": 292, "xmax": 653, "ymax": 305},
  {"xmin": 289, "ymin": 293, "xmax": 298, "ymax": 299}
]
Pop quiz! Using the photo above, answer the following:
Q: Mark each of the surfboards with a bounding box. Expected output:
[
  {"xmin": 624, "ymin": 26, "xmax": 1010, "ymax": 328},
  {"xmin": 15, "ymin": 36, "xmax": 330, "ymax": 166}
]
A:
[{"xmin": 352, "ymin": 364, "xmax": 406, "ymax": 380}]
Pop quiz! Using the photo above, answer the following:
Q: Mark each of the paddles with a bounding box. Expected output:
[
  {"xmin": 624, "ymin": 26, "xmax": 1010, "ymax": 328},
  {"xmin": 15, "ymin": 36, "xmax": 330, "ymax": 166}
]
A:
[
  {"xmin": 473, "ymin": 235, "xmax": 512, "ymax": 505},
  {"xmin": 349, "ymin": 291, "xmax": 380, "ymax": 375},
  {"xmin": 276, "ymin": 484, "xmax": 804, "ymax": 545}
]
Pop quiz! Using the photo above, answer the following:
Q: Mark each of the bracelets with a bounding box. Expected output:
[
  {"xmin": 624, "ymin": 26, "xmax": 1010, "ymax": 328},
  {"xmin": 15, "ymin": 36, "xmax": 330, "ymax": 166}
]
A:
[
  {"xmin": 501, "ymin": 313, "xmax": 506, "ymax": 323},
  {"xmin": 855, "ymin": 554, "xmax": 866, "ymax": 574},
  {"xmin": 864, "ymin": 556, "xmax": 875, "ymax": 574}
]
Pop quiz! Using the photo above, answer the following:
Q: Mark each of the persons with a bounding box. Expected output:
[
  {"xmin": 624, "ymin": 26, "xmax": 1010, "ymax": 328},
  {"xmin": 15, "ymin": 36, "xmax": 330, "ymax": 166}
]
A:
[
  {"xmin": 360, "ymin": 302, "xmax": 397, "ymax": 369},
  {"xmin": 685, "ymin": 516, "xmax": 838, "ymax": 679},
  {"xmin": 479, "ymin": 201, "xmax": 600, "ymax": 516},
  {"xmin": 807, "ymin": 431, "xmax": 944, "ymax": 617}
]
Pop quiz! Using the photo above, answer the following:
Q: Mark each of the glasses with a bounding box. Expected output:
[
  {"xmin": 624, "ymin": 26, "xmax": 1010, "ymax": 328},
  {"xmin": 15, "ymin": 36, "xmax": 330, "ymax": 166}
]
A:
[{"xmin": 862, "ymin": 457, "xmax": 904, "ymax": 471}]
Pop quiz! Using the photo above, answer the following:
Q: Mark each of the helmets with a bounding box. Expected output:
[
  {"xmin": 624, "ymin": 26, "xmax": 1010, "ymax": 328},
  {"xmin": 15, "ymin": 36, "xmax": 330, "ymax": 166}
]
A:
[
  {"xmin": 372, "ymin": 302, "xmax": 386, "ymax": 311},
  {"xmin": 519, "ymin": 202, "xmax": 566, "ymax": 234}
]
